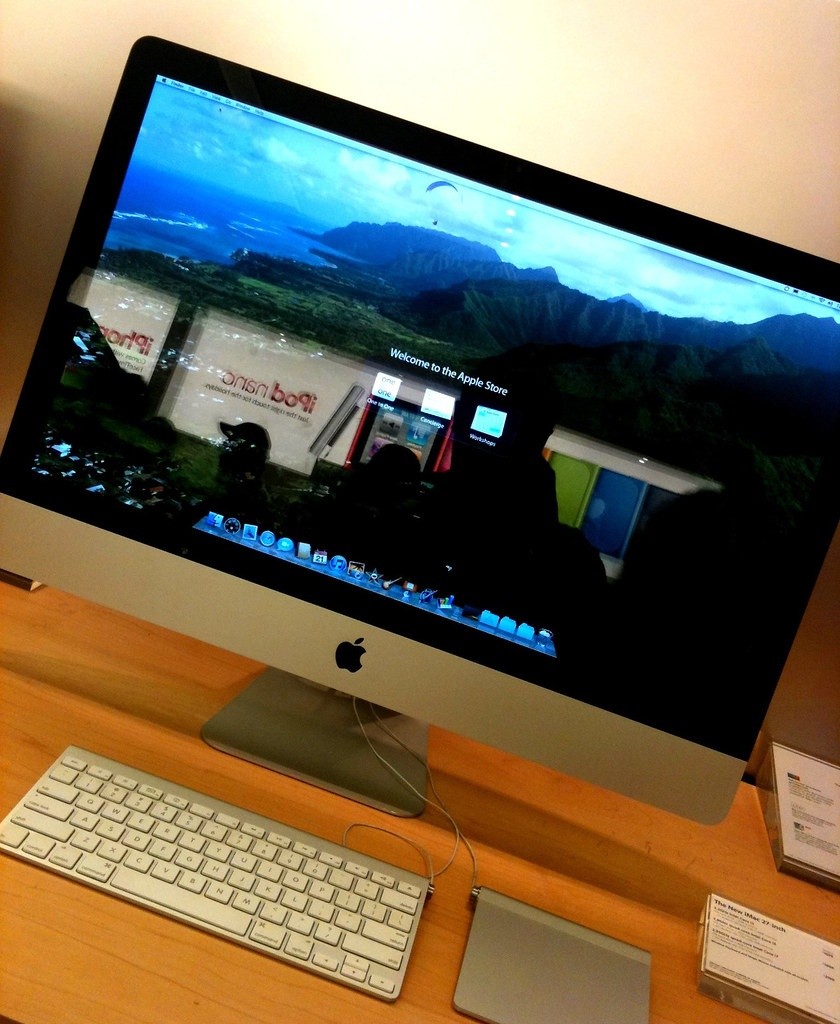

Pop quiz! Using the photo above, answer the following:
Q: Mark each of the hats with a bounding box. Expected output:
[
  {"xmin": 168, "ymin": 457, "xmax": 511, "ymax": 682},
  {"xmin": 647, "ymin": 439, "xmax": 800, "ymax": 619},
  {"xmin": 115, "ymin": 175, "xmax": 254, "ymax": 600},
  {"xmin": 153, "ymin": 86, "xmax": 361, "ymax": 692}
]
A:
[{"xmin": 219, "ymin": 422, "xmax": 269, "ymax": 458}]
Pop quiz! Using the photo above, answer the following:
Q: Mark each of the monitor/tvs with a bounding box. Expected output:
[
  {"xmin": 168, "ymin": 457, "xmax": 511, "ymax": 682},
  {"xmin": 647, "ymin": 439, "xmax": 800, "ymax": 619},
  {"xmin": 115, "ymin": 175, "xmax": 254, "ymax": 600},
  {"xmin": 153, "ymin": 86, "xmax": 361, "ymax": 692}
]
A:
[{"xmin": 0, "ymin": 37, "xmax": 840, "ymax": 822}]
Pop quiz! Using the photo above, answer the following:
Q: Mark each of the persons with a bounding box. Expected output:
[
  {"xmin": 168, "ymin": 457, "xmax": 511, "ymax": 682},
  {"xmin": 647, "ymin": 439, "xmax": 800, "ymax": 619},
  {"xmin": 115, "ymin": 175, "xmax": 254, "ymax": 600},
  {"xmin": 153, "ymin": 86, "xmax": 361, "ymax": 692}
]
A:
[{"xmin": 45, "ymin": 306, "xmax": 790, "ymax": 708}]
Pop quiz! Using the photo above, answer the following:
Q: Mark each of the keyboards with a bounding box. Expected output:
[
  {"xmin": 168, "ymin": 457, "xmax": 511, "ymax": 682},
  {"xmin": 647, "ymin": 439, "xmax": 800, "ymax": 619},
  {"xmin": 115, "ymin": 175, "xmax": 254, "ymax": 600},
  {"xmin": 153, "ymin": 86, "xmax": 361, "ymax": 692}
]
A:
[{"xmin": 1, "ymin": 746, "xmax": 433, "ymax": 1006}]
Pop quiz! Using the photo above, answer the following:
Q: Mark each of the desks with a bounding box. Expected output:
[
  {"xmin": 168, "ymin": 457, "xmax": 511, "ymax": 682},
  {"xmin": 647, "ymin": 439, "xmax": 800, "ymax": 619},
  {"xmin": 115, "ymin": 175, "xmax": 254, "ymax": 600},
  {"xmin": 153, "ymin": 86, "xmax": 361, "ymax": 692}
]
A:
[{"xmin": 0, "ymin": 571, "xmax": 840, "ymax": 1024}]
